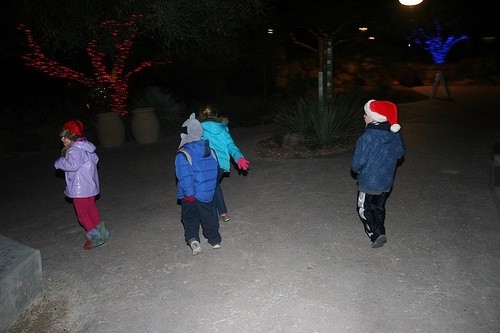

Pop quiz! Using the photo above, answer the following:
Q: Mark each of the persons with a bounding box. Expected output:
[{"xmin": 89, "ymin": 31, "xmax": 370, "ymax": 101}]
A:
[
  {"xmin": 175, "ymin": 113, "xmax": 222, "ymax": 256},
  {"xmin": 196, "ymin": 105, "xmax": 249, "ymax": 221},
  {"xmin": 54, "ymin": 119, "xmax": 110, "ymax": 250},
  {"xmin": 351, "ymin": 99, "xmax": 405, "ymax": 247}
]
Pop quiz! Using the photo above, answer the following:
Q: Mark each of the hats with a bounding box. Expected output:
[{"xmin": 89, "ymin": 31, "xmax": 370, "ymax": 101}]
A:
[
  {"xmin": 364, "ymin": 100, "xmax": 401, "ymax": 133},
  {"xmin": 63, "ymin": 120, "xmax": 84, "ymax": 137}
]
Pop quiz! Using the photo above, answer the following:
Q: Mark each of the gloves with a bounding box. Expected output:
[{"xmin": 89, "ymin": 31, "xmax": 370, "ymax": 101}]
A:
[{"xmin": 238, "ymin": 157, "xmax": 250, "ymax": 170}]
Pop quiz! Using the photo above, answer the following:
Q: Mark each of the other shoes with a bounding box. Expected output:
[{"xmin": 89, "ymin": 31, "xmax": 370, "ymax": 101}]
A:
[
  {"xmin": 220, "ymin": 212, "xmax": 230, "ymax": 222},
  {"xmin": 213, "ymin": 242, "xmax": 221, "ymax": 249},
  {"xmin": 190, "ymin": 241, "xmax": 202, "ymax": 255},
  {"xmin": 372, "ymin": 235, "xmax": 385, "ymax": 248}
]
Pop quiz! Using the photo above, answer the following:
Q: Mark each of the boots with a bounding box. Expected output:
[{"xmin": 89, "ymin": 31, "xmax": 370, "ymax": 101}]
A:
[
  {"xmin": 94, "ymin": 221, "xmax": 110, "ymax": 241},
  {"xmin": 84, "ymin": 228, "xmax": 105, "ymax": 248}
]
